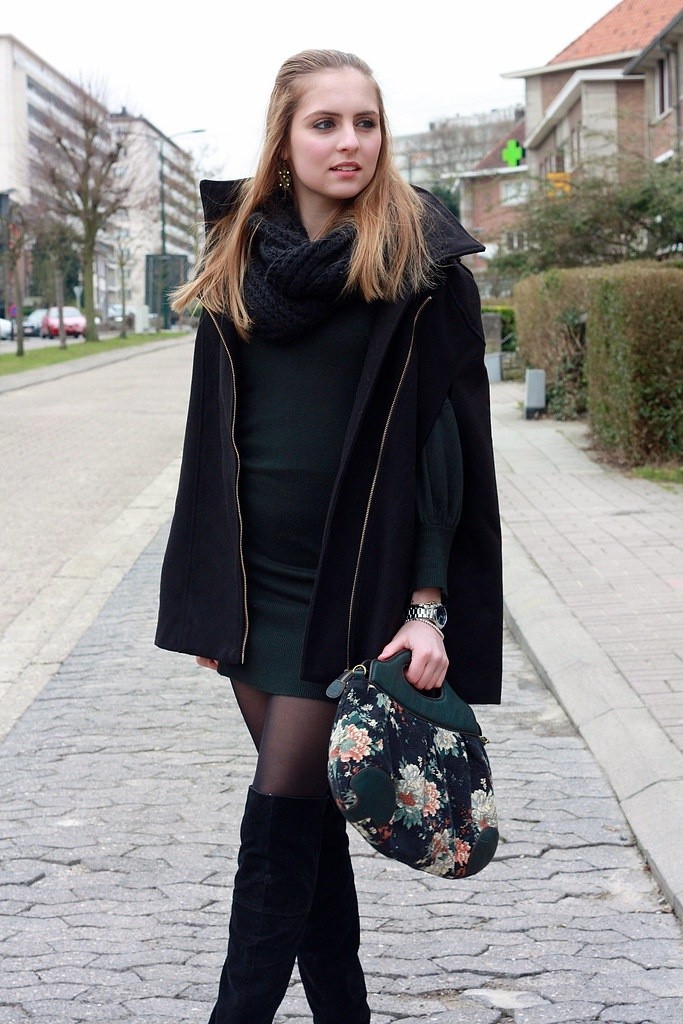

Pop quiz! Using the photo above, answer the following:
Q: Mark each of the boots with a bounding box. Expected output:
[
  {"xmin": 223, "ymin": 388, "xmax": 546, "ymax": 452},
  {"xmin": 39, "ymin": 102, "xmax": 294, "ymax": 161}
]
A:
[
  {"xmin": 298, "ymin": 788, "xmax": 371, "ymax": 1024},
  {"xmin": 208, "ymin": 786, "xmax": 336, "ymax": 1024}
]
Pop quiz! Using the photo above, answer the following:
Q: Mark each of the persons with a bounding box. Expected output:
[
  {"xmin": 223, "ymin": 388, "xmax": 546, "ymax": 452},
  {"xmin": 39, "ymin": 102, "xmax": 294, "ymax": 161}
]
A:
[{"xmin": 153, "ymin": 50, "xmax": 504, "ymax": 1024}]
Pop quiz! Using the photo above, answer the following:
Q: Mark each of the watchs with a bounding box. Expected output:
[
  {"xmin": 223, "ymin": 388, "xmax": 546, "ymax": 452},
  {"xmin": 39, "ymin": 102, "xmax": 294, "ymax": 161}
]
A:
[{"xmin": 405, "ymin": 602, "xmax": 447, "ymax": 629}]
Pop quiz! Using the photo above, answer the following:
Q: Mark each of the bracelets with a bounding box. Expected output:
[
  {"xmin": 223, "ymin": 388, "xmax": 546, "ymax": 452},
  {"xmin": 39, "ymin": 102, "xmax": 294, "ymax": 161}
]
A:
[{"xmin": 404, "ymin": 618, "xmax": 444, "ymax": 641}]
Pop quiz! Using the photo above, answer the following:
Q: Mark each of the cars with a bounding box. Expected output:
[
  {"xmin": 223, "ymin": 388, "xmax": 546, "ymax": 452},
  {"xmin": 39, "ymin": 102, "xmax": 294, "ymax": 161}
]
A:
[
  {"xmin": 0, "ymin": 317, "xmax": 17, "ymax": 342},
  {"xmin": 39, "ymin": 305, "xmax": 88, "ymax": 339},
  {"xmin": 19, "ymin": 308, "xmax": 50, "ymax": 337}
]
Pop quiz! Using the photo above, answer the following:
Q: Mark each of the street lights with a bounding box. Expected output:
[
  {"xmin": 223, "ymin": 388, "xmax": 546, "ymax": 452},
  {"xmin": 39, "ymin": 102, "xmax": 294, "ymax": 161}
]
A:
[{"xmin": 117, "ymin": 128, "xmax": 208, "ymax": 330}]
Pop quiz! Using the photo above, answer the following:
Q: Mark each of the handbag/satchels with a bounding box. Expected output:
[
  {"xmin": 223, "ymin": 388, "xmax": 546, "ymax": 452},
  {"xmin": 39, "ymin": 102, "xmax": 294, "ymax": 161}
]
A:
[{"xmin": 327, "ymin": 649, "xmax": 501, "ymax": 880}]
